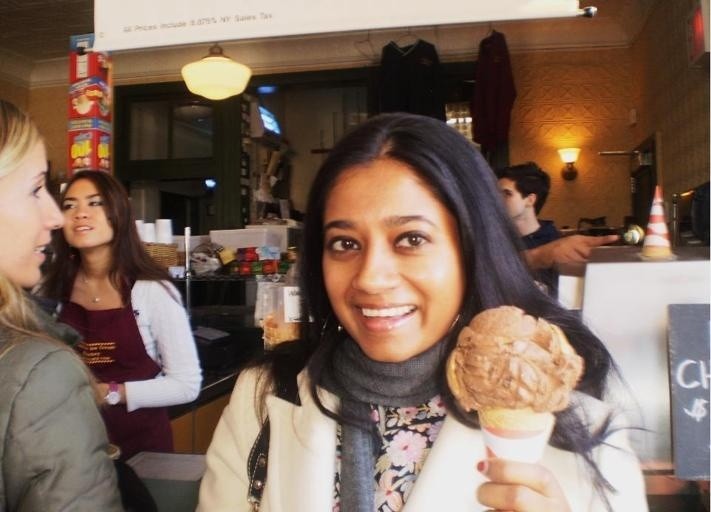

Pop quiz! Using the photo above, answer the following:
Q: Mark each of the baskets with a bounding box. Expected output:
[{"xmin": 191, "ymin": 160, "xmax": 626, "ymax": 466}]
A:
[{"xmin": 141, "ymin": 241, "xmax": 177, "ymax": 269}]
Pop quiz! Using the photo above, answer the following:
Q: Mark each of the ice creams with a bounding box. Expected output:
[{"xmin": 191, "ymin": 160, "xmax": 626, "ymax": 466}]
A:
[{"xmin": 445, "ymin": 305, "xmax": 584, "ymax": 466}]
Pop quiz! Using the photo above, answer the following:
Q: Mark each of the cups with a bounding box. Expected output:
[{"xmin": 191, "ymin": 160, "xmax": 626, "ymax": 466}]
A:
[{"xmin": 135, "ymin": 218, "xmax": 174, "ymax": 243}]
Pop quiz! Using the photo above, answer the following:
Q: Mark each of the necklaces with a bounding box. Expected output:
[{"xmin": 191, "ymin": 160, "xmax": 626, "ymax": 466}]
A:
[{"xmin": 79, "ymin": 265, "xmax": 111, "ymax": 303}]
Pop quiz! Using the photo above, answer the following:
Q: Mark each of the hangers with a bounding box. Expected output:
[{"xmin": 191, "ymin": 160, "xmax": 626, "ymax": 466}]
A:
[
  {"xmin": 484, "ymin": 22, "xmax": 496, "ymax": 38},
  {"xmin": 353, "ymin": 28, "xmax": 418, "ymax": 63}
]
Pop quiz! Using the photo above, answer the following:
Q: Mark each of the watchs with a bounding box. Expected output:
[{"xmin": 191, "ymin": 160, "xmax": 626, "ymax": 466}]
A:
[{"xmin": 103, "ymin": 379, "xmax": 121, "ymax": 406}]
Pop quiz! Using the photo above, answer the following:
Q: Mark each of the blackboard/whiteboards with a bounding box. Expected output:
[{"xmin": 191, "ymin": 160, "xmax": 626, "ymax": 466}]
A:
[{"xmin": 666, "ymin": 302, "xmax": 711, "ymax": 481}]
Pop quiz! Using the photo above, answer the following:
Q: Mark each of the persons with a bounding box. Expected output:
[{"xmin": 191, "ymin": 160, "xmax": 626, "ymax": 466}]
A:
[
  {"xmin": 195, "ymin": 114, "xmax": 653, "ymax": 512},
  {"xmin": 0, "ymin": 100, "xmax": 122, "ymax": 510},
  {"xmin": 493, "ymin": 160, "xmax": 562, "ymax": 300},
  {"xmin": 518, "ymin": 233, "xmax": 620, "ymax": 272},
  {"xmin": 43, "ymin": 169, "xmax": 204, "ymax": 454}
]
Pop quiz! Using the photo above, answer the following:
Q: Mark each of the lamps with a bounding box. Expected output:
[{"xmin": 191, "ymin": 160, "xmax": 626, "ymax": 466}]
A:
[
  {"xmin": 181, "ymin": 42, "xmax": 252, "ymax": 101},
  {"xmin": 558, "ymin": 148, "xmax": 581, "ymax": 181}
]
